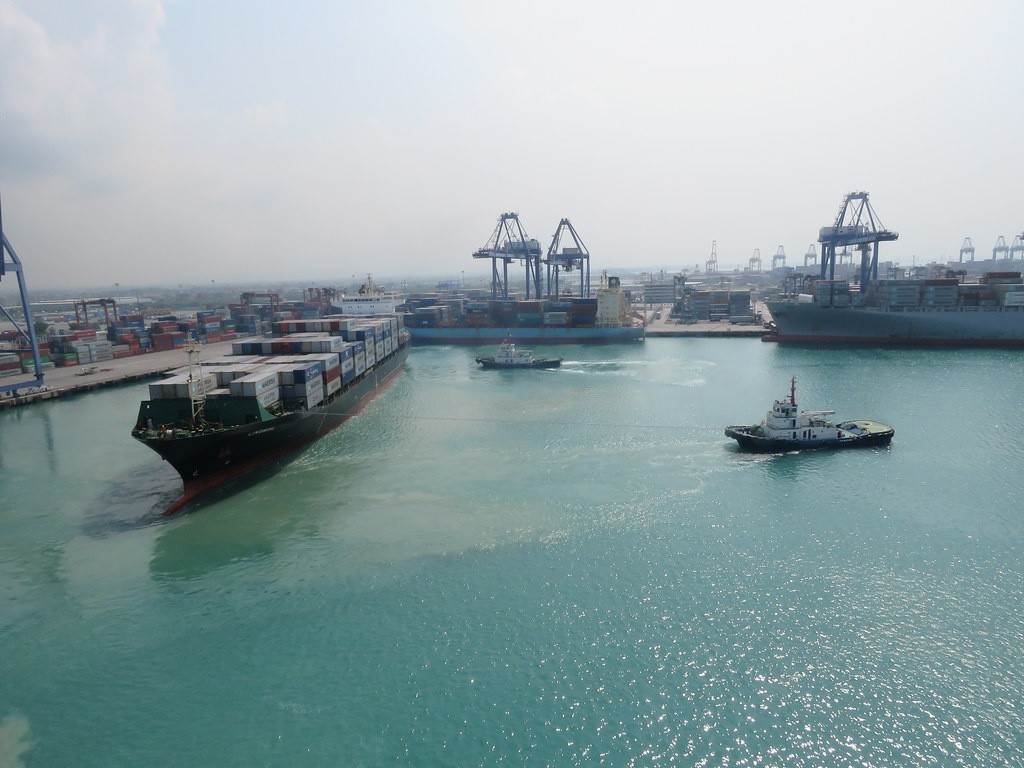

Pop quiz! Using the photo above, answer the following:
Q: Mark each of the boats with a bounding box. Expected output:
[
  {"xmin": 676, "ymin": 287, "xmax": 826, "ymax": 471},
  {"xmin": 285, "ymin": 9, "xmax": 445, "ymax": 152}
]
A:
[
  {"xmin": 475, "ymin": 343, "xmax": 564, "ymax": 370},
  {"xmin": 395, "ymin": 286, "xmax": 645, "ymax": 342},
  {"xmin": 130, "ymin": 314, "xmax": 410, "ymax": 485},
  {"xmin": 760, "ymin": 272, "xmax": 1024, "ymax": 347},
  {"xmin": 724, "ymin": 377, "xmax": 894, "ymax": 451}
]
